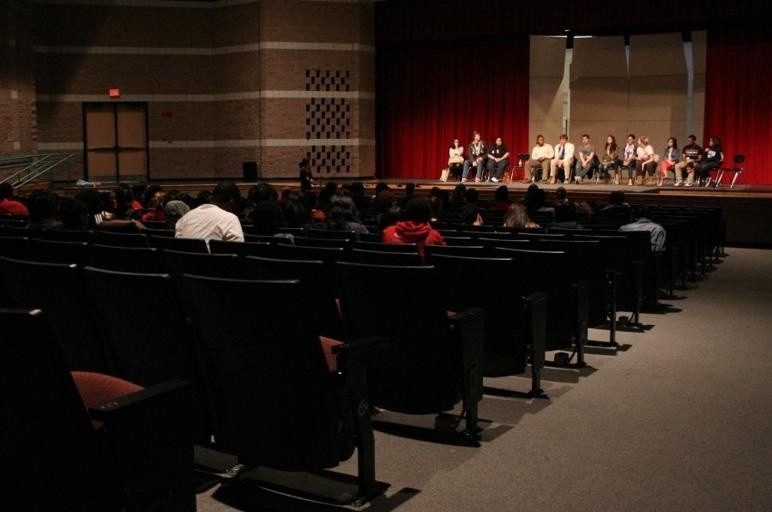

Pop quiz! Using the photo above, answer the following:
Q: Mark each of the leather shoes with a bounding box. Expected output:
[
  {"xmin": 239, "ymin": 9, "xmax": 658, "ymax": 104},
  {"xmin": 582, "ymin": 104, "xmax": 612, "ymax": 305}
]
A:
[{"xmin": 615, "ymin": 176, "xmax": 665, "ymax": 186}]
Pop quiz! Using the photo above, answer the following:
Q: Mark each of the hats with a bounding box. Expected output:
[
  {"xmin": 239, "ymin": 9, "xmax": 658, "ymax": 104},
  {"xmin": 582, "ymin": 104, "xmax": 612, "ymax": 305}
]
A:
[{"xmin": 165, "ymin": 200, "xmax": 191, "ymax": 219}]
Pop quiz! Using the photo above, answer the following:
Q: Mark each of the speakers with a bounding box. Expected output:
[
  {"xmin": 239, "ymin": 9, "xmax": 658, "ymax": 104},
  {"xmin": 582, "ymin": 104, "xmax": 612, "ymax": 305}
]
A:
[{"xmin": 243, "ymin": 162, "xmax": 257, "ymax": 180}]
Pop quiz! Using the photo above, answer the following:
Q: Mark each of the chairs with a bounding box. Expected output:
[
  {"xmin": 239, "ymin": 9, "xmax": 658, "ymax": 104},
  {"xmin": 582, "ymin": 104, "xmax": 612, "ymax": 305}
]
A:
[
  {"xmin": 451, "ymin": 145, "xmax": 747, "ymax": 189},
  {"xmin": 0, "ymin": 198, "xmax": 729, "ymax": 512}
]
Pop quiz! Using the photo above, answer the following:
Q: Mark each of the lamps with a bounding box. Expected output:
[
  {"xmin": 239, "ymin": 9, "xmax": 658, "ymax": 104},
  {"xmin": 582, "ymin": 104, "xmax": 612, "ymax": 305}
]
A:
[{"xmin": 565, "ymin": 26, "xmax": 574, "ymax": 49}]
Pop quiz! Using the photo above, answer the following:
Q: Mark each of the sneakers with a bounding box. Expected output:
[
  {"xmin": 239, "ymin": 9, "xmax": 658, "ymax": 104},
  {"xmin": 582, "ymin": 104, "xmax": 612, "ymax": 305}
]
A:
[
  {"xmin": 491, "ymin": 177, "xmax": 498, "ymax": 182},
  {"xmin": 674, "ymin": 181, "xmax": 683, "ymax": 186},
  {"xmin": 461, "ymin": 178, "xmax": 467, "ymax": 183},
  {"xmin": 519, "ymin": 175, "xmax": 581, "ymax": 184},
  {"xmin": 684, "ymin": 182, "xmax": 693, "ymax": 187},
  {"xmin": 475, "ymin": 177, "xmax": 480, "ymax": 183}
]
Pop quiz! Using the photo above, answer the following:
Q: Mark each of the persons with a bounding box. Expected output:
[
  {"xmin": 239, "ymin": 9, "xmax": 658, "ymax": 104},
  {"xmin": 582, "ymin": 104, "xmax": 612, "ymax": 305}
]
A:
[
  {"xmin": 2, "ymin": 157, "xmax": 666, "ymax": 252},
  {"xmin": 447, "ymin": 133, "xmax": 722, "ymax": 188}
]
